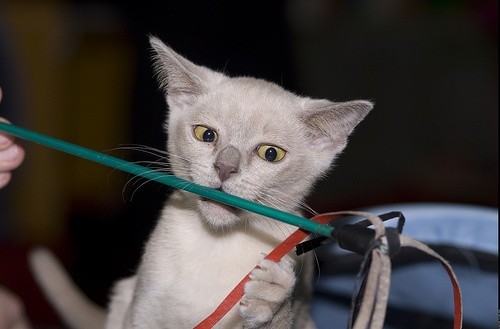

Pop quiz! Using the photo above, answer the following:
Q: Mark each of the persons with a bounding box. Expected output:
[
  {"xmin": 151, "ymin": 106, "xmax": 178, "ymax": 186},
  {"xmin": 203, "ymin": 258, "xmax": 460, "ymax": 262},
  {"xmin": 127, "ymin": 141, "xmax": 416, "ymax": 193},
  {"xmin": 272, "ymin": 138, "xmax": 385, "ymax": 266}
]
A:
[{"xmin": 0, "ymin": 115, "xmax": 32, "ymax": 329}]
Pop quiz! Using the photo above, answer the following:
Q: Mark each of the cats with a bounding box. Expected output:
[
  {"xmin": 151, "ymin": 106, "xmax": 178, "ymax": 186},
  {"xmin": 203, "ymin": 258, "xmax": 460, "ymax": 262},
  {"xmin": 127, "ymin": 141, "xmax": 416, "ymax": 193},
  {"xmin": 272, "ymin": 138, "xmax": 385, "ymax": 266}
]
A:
[{"xmin": 24, "ymin": 33, "xmax": 373, "ymax": 329}]
